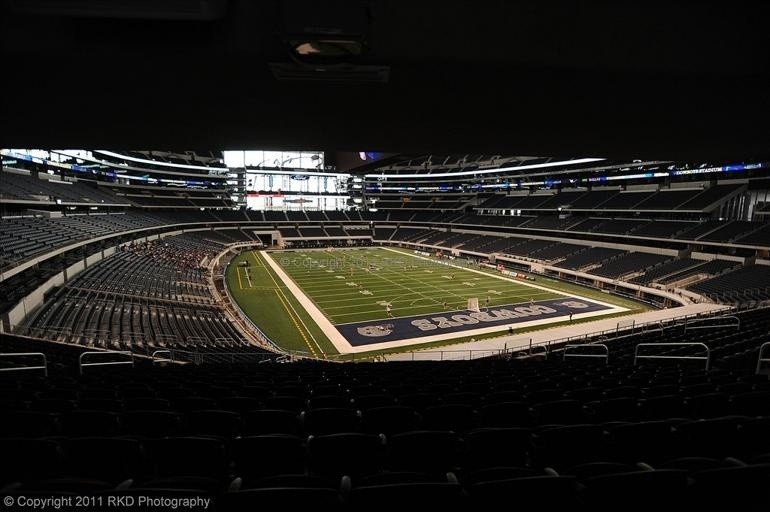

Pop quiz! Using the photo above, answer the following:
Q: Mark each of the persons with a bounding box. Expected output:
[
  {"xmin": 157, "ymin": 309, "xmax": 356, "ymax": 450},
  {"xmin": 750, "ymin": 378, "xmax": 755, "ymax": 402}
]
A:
[
  {"xmin": 386, "ymin": 304, "xmax": 394, "ymax": 318},
  {"xmin": 120, "ymin": 238, "xmax": 217, "ymax": 271},
  {"xmin": 484, "ymin": 297, "xmax": 490, "ymax": 307},
  {"xmin": 443, "ymin": 300, "xmax": 447, "ymax": 310},
  {"xmin": 530, "ymin": 298, "xmax": 535, "ymax": 306},
  {"xmin": 568, "ymin": 310, "xmax": 574, "ymax": 320},
  {"xmin": 509, "ymin": 327, "xmax": 513, "ymax": 335}
]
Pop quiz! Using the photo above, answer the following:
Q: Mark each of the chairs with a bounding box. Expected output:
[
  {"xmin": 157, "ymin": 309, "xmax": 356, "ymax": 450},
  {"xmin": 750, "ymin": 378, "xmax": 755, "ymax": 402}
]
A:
[
  {"xmin": 515, "ymin": 324, "xmax": 688, "ymax": 359},
  {"xmin": 249, "ymin": 346, "xmax": 290, "ymax": 359},
  {"xmin": 250, "ymin": 210, "xmax": 365, "ymax": 248},
  {"xmin": 687, "ymin": 286, "xmax": 770, "ymax": 358}
]
